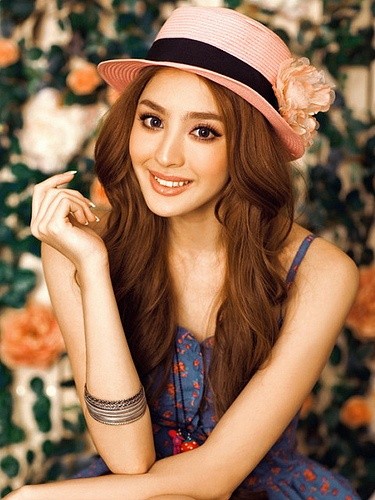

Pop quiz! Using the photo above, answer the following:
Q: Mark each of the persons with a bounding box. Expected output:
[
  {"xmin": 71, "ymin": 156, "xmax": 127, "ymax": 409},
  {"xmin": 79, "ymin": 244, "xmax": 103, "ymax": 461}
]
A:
[{"xmin": 0, "ymin": 5, "xmax": 361, "ymax": 500}]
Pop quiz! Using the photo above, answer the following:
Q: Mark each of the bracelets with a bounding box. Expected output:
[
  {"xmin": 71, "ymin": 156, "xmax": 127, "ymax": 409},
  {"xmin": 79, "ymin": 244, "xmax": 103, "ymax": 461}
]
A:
[{"xmin": 83, "ymin": 385, "xmax": 148, "ymax": 425}]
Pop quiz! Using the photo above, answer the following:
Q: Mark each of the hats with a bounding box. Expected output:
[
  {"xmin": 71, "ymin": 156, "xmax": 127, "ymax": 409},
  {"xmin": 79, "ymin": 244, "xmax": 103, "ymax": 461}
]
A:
[{"xmin": 97, "ymin": 7, "xmax": 336, "ymax": 159}]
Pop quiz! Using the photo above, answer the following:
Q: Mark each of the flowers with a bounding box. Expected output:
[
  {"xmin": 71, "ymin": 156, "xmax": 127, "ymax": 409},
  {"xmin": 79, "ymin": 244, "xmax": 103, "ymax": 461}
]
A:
[{"xmin": 271, "ymin": 57, "xmax": 338, "ymax": 145}]
